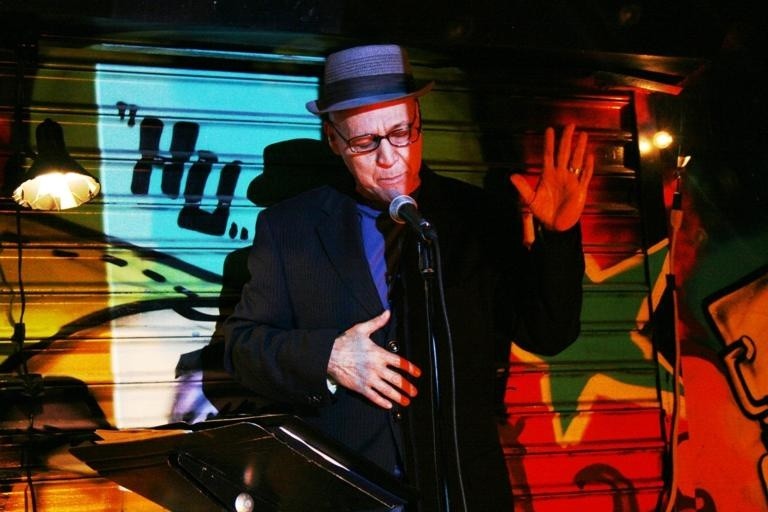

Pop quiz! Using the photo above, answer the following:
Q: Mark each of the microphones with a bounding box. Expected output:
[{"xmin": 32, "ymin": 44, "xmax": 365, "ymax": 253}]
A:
[{"xmin": 389, "ymin": 194, "xmax": 430, "ymax": 234}]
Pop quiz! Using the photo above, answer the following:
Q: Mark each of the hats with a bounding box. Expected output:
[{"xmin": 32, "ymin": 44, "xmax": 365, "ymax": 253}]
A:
[{"xmin": 306, "ymin": 43, "xmax": 436, "ymax": 120}]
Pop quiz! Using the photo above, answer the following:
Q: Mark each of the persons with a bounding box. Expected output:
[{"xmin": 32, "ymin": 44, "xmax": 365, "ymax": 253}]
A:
[{"xmin": 223, "ymin": 44, "xmax": 595, "ymax": 512}]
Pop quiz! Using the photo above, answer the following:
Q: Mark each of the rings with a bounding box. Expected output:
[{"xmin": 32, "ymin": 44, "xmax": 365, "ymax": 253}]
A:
[{"xmin": 568, "ymin": 166, "xmax": 582, "ymax": 176}]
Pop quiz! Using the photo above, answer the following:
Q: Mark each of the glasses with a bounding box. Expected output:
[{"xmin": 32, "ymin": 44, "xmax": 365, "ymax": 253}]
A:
[{"xmin": 322, "ymin": 98, "xmax": 422, "ymax": 156}]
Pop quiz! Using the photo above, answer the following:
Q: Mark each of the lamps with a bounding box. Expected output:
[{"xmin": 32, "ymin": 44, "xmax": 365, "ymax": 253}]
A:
[
  {"xmin": 651, "ymin": 129, "xmax": 674, "ymax": 150},
  {"xmin": 9, "ymin": 114, "xmax": 102, "ymax": 375}
]
